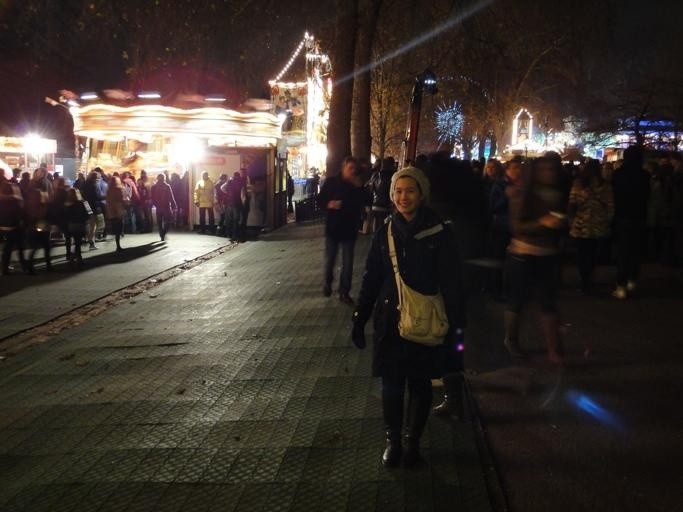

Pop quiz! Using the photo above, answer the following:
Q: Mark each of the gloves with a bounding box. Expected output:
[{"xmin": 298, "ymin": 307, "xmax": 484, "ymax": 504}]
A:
[{"xmin": 351, "ymin": 320, "xmax": 367, "ymax": 350}]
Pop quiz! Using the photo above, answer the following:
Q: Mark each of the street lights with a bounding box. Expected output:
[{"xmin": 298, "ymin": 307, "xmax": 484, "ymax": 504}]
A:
[{"xmin": 398, "ymin": 64, "xmax": 442, "ymax": 179}]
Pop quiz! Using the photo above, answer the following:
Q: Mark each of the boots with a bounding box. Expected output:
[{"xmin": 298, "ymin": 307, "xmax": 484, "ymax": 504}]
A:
[
  {"xmin": 502, "ymin": 310, "xmax": 525, "ymax": 359},
  {"xmin": 434, "ymin": 392, "xmax": 463, "ymax": 417},
  {"xmin": 381, "ymin": 428, "xmax": 404, "ymax": 465},
  {"xmin": 540, "ymin": 311, "xmax": 566, "ymax": 366}
]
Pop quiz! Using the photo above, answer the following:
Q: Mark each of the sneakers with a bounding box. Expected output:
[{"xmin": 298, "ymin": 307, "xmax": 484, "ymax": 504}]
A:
[
  {"xmin": 323, "ymin": 275, "xmax": 333, "ymax": 298},
  {"xmin": 336, "ymin": 293, "xmax": 353, "ymax": 304},
  {"xmin": 0, "ymin": 257, "xmax": 83, "ymax": 276},
  {"xmin": 403, "ymin": 432, "xmax": 420, "ymax": 468},
  {"xmin": 89, "ymin": 246, "xmax": 99, "ymax": 251}
]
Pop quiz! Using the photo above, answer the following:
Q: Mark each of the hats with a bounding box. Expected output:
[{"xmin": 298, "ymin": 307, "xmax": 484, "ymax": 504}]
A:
[{"xmin": 388, "ymin": 166, "xmax": 431, "ymax": 201}]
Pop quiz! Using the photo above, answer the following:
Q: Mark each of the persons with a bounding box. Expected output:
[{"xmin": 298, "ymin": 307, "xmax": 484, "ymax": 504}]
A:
[
  {"xmin": 0, "ymin": 162, "xmax": 295, "ymax": 276},
  {"xmin": 303, "ymin": 144, "xmax": 683, "ymax": 470}
]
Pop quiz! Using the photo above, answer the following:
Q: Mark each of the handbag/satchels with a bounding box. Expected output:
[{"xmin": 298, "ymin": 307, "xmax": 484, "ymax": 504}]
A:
[{"xmin": 397, "ymin": 279, "xmax": 450, "ymax": 349}]
